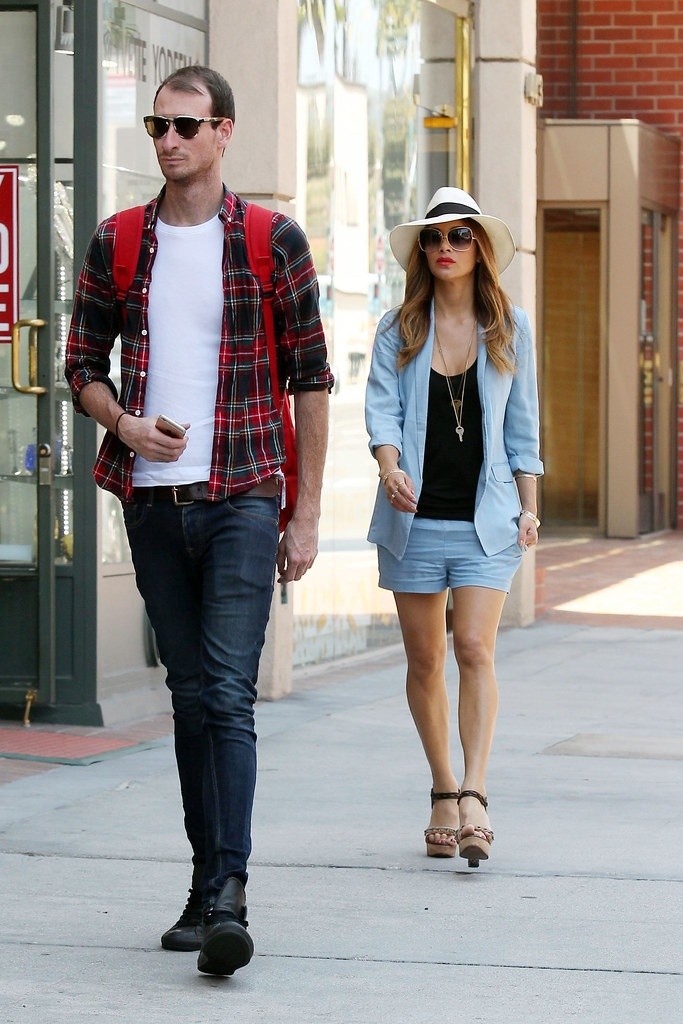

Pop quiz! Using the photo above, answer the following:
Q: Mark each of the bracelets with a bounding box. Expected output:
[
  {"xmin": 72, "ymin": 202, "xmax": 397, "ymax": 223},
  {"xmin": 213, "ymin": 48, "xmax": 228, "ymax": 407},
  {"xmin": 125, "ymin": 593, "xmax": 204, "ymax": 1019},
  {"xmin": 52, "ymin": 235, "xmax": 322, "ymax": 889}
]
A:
[
  {"xmin": 383, "ymin": 469, "xmax": 406, "ymax": 485},
  {"xmin": 515, "ymin": 474, "xmax": 537, "ymax": 482},
  {"xmin": 520, "ymin": 510, "xmax": 540, "ymax": 528},
  {"xmin": 115, "ymin": 412, "xmax": 129, "ymax": 439}
]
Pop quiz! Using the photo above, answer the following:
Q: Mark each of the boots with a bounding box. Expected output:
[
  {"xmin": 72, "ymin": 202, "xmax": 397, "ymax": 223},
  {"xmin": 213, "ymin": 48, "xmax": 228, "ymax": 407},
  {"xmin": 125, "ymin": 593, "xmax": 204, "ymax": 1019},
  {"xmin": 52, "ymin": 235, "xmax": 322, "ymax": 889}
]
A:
[
  {"xmin": 197, "ymin": 877, "xmax": 253, "ymax": 975},
  {"xmin": 161, "ymin": 855, "xmax": 205, "ymax": 952}
]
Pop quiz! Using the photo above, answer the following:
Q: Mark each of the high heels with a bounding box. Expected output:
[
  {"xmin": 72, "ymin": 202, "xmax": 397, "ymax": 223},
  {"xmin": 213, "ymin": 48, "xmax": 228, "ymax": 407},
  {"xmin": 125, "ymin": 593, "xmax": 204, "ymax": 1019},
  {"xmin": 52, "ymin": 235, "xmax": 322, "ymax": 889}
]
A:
[
  {"xmin": 424, "ymin": 788, "xmax": 459, "ymax": 857},
  {"xmin": 457, "ymin": 790, "xmax": 494, "ymax": 868}
]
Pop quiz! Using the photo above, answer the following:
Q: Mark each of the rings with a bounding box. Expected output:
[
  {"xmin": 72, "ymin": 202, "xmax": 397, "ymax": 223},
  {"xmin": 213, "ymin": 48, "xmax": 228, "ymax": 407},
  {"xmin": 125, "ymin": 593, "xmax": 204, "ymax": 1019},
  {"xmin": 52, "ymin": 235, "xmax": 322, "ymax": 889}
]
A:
[
  {"xmin": 390, "ymin": 482, "xmax": 405, "ymax": 504},
  {"xmin": 524, "ymin": 544, "xmax": 529, "ymax": 551}
]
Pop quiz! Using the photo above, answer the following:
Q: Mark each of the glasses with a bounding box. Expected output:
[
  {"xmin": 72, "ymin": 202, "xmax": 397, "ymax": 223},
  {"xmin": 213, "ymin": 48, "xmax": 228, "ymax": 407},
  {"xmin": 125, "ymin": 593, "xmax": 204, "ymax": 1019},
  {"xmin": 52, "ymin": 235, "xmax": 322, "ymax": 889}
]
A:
[
  {"xmin": 416, "ymin": 226, "xmax": 476, "ymax": 252},
  {"xmin": 143, "ymin": 115, "xmax": 225, "ymax": 139}
]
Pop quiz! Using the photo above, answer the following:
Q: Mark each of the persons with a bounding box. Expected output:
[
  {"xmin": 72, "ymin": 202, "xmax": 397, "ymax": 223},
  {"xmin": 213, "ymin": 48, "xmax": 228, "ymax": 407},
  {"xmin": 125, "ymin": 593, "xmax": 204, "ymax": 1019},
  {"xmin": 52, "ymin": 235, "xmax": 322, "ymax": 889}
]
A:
[
  {"xmin": 366, "ymin": 189, "xmax": 545, "ymax": 867},
  {"xmin": 64, "ymin": 66, "xmax": 334, "ymax": 976}
]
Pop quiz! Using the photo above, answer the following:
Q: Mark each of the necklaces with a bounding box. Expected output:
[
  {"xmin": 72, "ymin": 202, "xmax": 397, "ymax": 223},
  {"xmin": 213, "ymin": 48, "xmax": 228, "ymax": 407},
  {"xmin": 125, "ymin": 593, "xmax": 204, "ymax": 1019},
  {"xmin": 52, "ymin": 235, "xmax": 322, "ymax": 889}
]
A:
[{"xmin": 434, "ymin": 317, "xmax": 479, "ymax": 442}]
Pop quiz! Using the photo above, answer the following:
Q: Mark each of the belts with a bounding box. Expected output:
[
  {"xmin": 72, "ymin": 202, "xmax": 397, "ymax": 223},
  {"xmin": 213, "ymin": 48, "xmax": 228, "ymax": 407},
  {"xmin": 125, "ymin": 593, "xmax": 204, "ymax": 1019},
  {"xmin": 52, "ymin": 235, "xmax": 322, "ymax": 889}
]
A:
[{"xmin": 132, "ymin": 479, "xmax": 279, "ymax": 504}]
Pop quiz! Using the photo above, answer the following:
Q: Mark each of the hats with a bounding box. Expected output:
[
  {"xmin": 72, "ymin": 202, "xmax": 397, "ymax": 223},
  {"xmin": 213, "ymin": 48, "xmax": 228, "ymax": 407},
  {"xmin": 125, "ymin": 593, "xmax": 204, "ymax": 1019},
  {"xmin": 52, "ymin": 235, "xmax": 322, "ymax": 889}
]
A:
[{"xmin": 390, "ymin": 186, "xmax": 515, "ymax": 277}]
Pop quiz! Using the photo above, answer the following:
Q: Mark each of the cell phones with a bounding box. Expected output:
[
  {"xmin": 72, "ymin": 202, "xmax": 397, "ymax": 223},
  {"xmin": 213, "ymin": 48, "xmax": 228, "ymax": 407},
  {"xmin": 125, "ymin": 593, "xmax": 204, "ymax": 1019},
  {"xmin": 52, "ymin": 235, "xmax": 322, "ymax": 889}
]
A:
[{"xmin": 155, "ymin": 414, "xmax": 186, "ymax": 440}]
